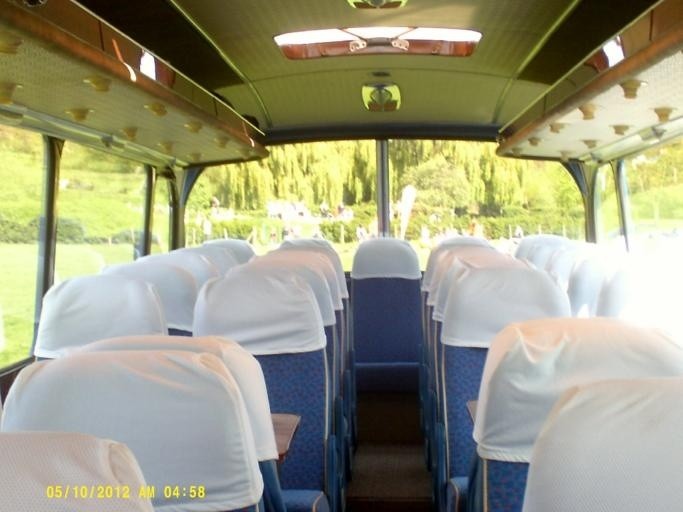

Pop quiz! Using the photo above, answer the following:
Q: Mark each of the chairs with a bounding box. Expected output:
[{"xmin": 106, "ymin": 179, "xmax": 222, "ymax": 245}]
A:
[{"xmin": 351, "ymin": 239, "xmax": 420, "ymax": 392}]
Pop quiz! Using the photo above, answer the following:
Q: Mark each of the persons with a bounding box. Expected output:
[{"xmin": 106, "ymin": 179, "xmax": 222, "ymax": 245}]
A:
[{"xmin": 210, "ymin": 197, "xmax": 221, "ymax": 209}]
